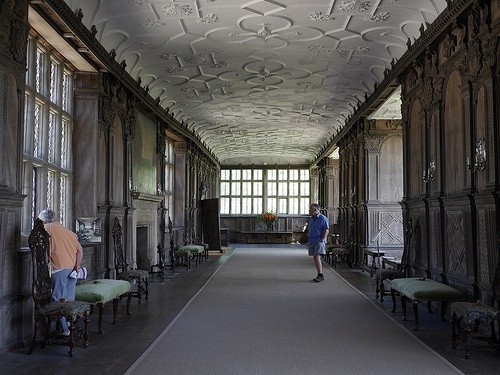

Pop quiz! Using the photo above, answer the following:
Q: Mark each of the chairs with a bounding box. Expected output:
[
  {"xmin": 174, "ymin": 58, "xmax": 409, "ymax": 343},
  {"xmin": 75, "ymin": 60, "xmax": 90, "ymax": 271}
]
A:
[
  {"xmin": 26, "ymin": 218, "xmax": 91, "ymax": 357},
  {"xmin": 375, "ymin": 216, "xmax": 415, "ymax": 303},
  {"xmin": 450, "ymin": 242, "xmax": 500, "ymax": 359},
  {"xmin": 191, "ymin": 214, "xmax": 209, "ymax": 262},
  {"xmin": 112, "ymin": 215, "xmax": 149, "ymax": 304},
  {"xmin": 168, "ymin": 216, "xmax": 191, "ymax": 270}
]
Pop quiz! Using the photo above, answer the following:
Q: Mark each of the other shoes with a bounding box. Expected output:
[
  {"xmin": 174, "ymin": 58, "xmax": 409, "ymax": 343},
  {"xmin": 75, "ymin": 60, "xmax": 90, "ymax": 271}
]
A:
[
  {"xmin": 57, "ymin": 330, "xmax": 70, "ymax": 336},
  {"xmin": 67, "ymin": 322, "xmax": 70, "ymax": 327},
  {"xmin": 312, "ymin": 275, "xmax": 324, "ymax": 282}
]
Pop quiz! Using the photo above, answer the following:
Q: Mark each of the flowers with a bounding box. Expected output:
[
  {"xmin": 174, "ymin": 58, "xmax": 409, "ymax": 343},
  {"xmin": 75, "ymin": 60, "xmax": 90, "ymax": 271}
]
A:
[{"xmin": 257, "ymin": 210, "xmax": 279, "ymax": 226}]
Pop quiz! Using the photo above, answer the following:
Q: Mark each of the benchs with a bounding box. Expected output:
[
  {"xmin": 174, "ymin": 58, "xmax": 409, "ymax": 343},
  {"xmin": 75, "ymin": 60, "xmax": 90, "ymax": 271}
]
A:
[
  {"xmin": 179, "ymin": 244, "xmax": 204, "ymax": 267},
  {"xmin": 389, "ymin": 277, "xmax": 461, "ymax": 331},
  {"xmin": 75, "ymin": 279, "xmax": 135, "ymax": 334}
]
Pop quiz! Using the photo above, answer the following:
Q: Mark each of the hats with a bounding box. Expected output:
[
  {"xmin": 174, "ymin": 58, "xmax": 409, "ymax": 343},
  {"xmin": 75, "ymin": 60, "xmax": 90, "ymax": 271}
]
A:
[{"xmin": 39, "ymin": 209, "xmax": 57, "ymax": 222}]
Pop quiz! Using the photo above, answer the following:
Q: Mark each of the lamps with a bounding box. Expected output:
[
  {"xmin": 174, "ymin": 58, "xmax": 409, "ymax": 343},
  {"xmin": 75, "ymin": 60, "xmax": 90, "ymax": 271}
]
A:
[
  {"xmin": 422, "ymin": 156, "xmax": 437, "ymax": 182},
  {"xmin": 465, "ymin": 137, "xmax": 487, "ymax": 173}
]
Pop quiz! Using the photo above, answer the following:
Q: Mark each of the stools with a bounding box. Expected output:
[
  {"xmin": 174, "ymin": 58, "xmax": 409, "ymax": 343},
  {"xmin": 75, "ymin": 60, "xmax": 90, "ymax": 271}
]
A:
[
  {"xmin": 362, "ymin": 250, "xmax": 385, "ymax": 277},
  {"xmin": 219, "ymin": 227, "xmax": 230, "ymax": 246},
  {"xmin": 380, "ymin": 256, "xmax": 403, "ymax": 271}
]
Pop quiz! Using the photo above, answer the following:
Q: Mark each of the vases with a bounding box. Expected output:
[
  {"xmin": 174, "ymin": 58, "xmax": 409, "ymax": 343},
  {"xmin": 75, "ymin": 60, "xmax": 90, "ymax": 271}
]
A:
[{"xmin": 267, "ymin": 223, "xmax": 273, "ymax": 232}]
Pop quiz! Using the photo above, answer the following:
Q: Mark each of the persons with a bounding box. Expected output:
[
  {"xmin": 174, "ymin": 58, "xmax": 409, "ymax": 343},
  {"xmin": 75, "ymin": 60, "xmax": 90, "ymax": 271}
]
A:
[
  {"xmin": 307, "ymin": 204, "xmax": 329, "ymax": 282},
  {"xmin": 39, "ymin": 209, "xmax": 84, "ymax": 340}
]
